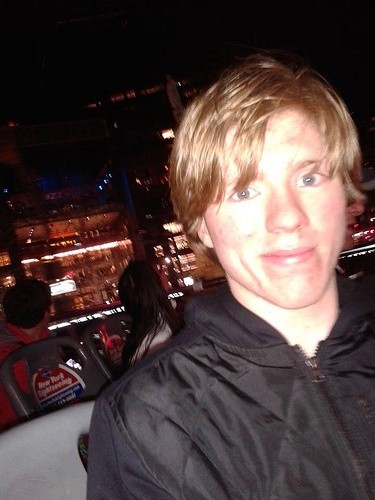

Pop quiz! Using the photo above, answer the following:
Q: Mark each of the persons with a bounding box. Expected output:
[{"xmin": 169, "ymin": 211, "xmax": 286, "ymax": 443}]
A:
[
  {"xmin": 87, "ymin": 55, "xmax": 375, "ymax": 500},
  {"xmin": 118, "ymin": 261, "xmax": 181, "ymax": 376},
  {"xmin": 0, "ymin": 278, "xmax": 57, "ymax": 434}
]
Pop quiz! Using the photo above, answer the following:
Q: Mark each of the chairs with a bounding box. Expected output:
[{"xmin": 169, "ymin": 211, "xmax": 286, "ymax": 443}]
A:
[
  {"xmin": 0, "ymin": 399, "xmax": 95, "ymax": 500},
  {"xmin": 82, "ymin": 312, "xmax": 132, "ymax": 383},
  {"xmin": 0, "ymin": 335, "xmax": 110, "ymax": 421}
]
